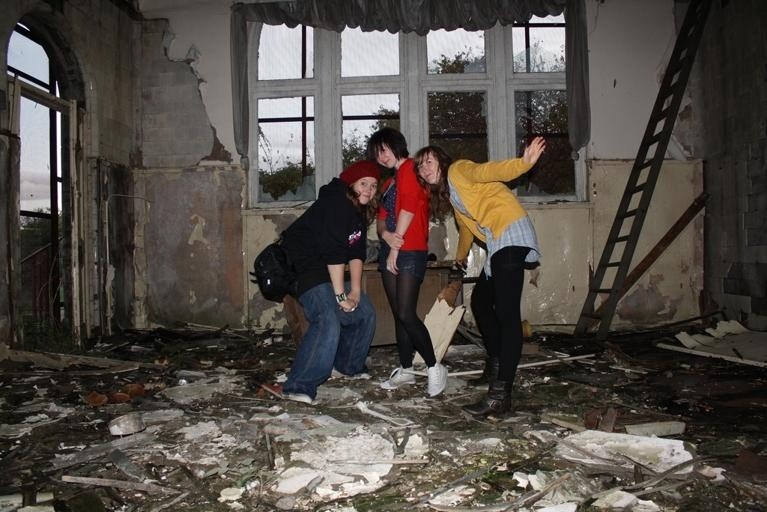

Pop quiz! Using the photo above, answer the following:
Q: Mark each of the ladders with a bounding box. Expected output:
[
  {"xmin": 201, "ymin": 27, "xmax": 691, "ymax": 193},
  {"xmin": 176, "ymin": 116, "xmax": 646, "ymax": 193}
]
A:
[{"xmin": 569, "ymin": 0, "xmax": 712, "ymax": 343}]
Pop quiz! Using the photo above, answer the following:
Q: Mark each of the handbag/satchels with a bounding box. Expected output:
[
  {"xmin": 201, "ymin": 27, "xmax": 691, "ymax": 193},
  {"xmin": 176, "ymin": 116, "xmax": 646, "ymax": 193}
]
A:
[{"xmin": 249, "ymin": 244, "xmax": 300, "ymax": 303}]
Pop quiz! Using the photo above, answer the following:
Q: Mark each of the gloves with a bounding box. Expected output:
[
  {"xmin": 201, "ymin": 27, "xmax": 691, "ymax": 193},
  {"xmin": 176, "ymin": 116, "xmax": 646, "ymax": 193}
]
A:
[{"xmin": 437, "ymin": 260, "xmax": 468, "ymax": 308}]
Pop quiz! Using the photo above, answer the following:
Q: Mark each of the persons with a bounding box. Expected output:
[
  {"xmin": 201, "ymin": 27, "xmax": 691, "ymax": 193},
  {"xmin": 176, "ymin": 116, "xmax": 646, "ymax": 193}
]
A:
[
  {"xmin": 274, "ymin": 160, "xmax": 376, "ymax": 405},
  {"xmin": 414, "ymin": 137, "xmax": 546, "ymax": 416},
  {"xmin": 365, "ymin": 127, "xmax": 448, "ymax": 397}
]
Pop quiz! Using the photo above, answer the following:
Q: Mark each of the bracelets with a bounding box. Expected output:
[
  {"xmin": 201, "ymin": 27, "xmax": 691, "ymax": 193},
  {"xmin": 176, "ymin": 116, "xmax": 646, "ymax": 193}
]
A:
[{"xmin": 334, "ymin": 292, "xmax": 347, "ymax": 303}]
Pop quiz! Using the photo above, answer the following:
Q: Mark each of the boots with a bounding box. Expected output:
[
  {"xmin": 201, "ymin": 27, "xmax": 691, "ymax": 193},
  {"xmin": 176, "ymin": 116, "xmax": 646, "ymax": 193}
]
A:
[
  {"xmin": 463, "ymin": 380, "xmax": 513, "ymax": 418},
  {"xmin": 467, "ymin": 357, "xmax": 494, "ymax": 386}
]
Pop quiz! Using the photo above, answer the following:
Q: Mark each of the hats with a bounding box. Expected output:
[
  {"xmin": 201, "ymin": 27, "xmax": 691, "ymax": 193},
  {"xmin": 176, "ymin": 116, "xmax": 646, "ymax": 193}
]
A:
[{"xmin": 340, "ymin": 161, "xmax": 380, "ymax": 188}]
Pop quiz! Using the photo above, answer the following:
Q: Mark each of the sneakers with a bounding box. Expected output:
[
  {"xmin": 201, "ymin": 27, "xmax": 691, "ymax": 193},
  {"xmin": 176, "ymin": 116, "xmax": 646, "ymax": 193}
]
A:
[
  {"xmin": 427, "ymin": 364, "xmax": 448, "ymax": 397},
  {"xmin": 382, "ymin": 366, "xmax": 414, "ymax": 389},
  {"xmin": 331, "ymin": 368, "xmax": 370, "ymax": 380},
  {"xmin": 281, "ymin": 391, "xmax": 313, "ymax": 405}
]
